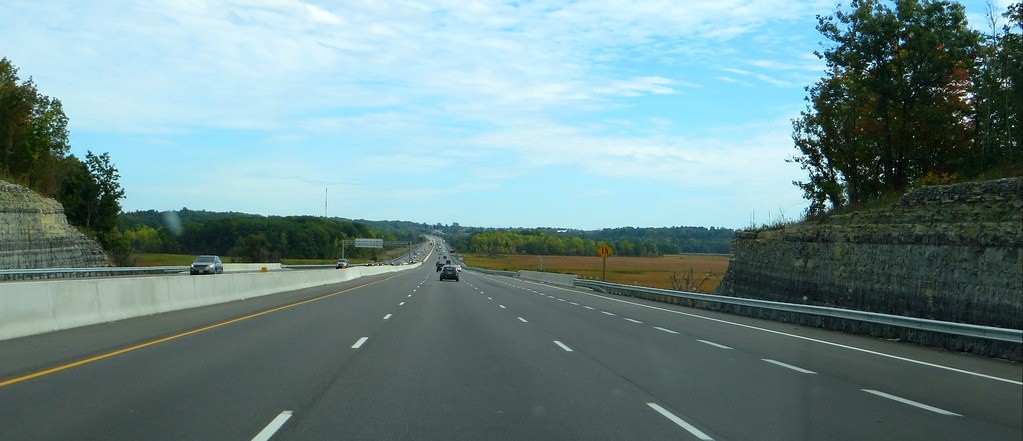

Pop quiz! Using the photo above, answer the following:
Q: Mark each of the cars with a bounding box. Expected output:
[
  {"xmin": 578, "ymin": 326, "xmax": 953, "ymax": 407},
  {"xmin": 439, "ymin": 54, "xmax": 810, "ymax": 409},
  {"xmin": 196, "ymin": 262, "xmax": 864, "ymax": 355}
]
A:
[
  {"xmin": 367, "ymin": 259, "xmax": 461, "ymax": 272},
  {"xmin": 335, "ymin": 258, "xmax": 349, "ymax": 269},
  {"xmin": 190, "ymin": 255, "xmax": 223, "ymax": 275},
  {"xmin": 440, "ymin": 265, "xmax": 459, "ymax": 282}
]
